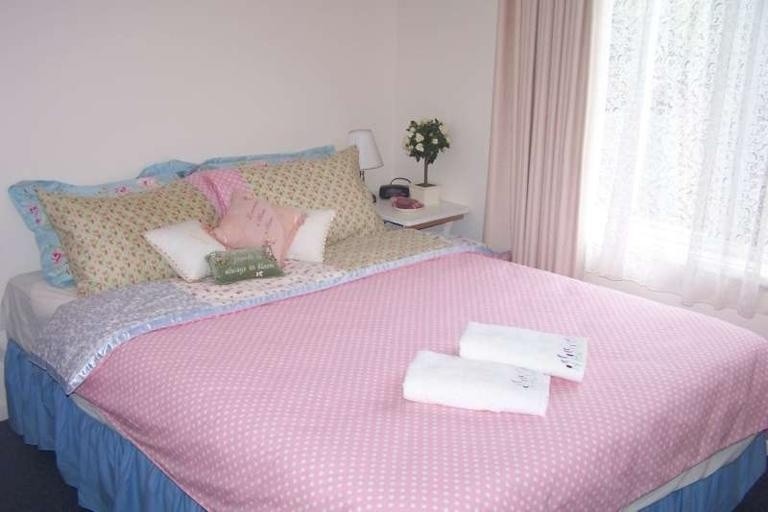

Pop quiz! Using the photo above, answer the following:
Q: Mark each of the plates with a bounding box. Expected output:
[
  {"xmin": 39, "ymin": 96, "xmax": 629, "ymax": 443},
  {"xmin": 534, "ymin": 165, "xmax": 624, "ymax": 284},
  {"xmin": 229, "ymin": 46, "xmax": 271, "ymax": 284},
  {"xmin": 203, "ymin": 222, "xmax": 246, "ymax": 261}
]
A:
[{"xmin": 390, "ymin": 196, "xmax": 429, "ymax": 214}]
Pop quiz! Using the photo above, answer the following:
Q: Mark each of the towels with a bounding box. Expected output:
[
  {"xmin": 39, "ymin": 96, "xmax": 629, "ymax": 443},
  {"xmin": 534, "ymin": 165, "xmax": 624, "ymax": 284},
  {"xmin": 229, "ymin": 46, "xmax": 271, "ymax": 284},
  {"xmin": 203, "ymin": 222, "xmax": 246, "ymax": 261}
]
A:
[
  {"xmin": 458, "ymin": 320, "xmax": 588, "ymax": 384},
  {"xmin": 401, "ymin": 350, "xmax": 551, "ymax": 419}
]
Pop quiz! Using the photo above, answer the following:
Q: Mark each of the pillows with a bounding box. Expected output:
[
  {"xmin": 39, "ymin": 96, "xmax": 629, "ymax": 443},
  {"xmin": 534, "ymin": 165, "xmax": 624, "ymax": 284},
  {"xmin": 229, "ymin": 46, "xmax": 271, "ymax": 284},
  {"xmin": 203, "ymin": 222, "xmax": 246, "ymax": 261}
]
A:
[
  {"xmin": 140, "ymin": 220, "xmax": 228, "ymax": 282},
  {"xmin": 8, "ymin": 151, "xmax": 184, "ymax": 291},
  {"xmin": 207, "ymin": 187, "xmax": 307, "ymax": 269},
  {"xmin": 287, "ymin": 210, "xmax": 330, "ymax": 264},
  {"xmin": 190, "ymin": 145, "xmax": 332, "ymax": 172},
  {"xmin": 204, "ymin": 238, "xmax": 284, "ymax": 281},
  {"xmin": 193, "ymin": 144, "xmax": 376, "ymax": 247},
  {"xmin": 35, "ymin": 173, "xmax": 227, "ymax": 299}
]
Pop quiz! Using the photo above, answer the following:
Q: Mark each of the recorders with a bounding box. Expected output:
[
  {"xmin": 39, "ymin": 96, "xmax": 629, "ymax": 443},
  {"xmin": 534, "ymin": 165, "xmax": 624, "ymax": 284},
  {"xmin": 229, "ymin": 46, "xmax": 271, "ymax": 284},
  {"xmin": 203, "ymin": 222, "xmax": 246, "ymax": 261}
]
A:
[{"xmin": 379, "ymin": 184, "xmax": 409, "ymax": 199}]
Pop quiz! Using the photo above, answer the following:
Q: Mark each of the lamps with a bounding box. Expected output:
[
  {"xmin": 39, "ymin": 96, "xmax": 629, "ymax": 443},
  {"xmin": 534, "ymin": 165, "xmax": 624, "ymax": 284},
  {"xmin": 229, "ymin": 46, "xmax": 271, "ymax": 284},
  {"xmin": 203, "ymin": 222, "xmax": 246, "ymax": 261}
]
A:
[{"xmin": 347, "ymin": 129, "xmax": 384, "ymax": 203}]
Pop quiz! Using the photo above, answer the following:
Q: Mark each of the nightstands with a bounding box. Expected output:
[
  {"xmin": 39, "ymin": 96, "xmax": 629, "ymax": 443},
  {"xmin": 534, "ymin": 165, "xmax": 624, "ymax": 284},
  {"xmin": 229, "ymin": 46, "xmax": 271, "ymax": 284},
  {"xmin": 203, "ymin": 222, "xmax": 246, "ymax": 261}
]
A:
[{"xmin": 375, "ymin": 194, "xmax": 472, "ymax": 231}]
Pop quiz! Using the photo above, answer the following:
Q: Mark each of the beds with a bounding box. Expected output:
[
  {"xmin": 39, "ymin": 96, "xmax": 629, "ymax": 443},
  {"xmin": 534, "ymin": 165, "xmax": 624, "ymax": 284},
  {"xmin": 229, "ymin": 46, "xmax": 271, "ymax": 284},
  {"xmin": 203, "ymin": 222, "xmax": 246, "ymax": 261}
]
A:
[{"xmin": 1, "ymin": 221, "xmax": 767, "ymax": 512}]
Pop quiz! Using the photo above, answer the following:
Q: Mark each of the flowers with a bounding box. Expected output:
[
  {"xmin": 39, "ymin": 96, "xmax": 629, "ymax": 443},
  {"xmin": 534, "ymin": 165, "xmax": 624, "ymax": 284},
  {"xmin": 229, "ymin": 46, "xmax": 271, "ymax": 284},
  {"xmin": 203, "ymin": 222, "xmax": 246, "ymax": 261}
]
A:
[{"xmin": 400, "ymin": 116, "xmax": 453, "ymax": 184}]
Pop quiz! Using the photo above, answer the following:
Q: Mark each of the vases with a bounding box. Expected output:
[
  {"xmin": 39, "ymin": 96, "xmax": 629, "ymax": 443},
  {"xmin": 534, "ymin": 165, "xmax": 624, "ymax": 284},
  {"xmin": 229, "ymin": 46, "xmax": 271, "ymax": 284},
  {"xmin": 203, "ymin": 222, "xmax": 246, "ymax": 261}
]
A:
[{"xmin": 409, "ymin": 184, "xmax": 441, "ymax": 209}]
